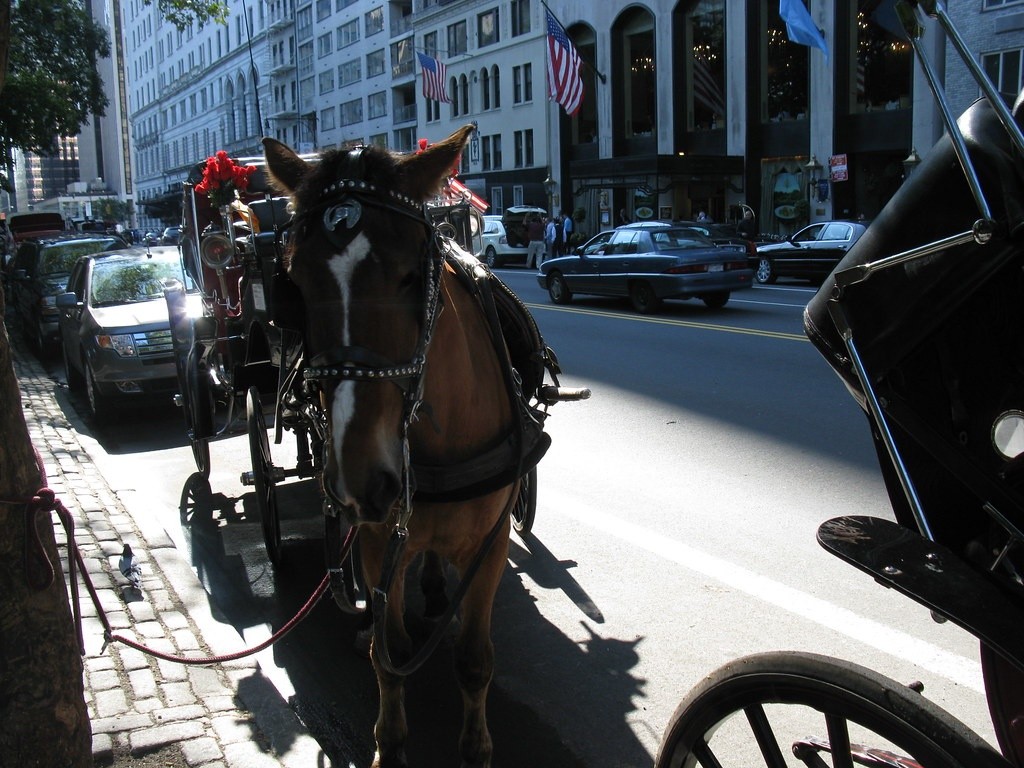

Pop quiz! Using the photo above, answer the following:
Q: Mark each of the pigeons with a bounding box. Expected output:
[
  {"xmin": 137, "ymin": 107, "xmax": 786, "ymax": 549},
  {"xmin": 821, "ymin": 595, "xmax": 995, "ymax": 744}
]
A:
[{"xmin": 118, "ymin": 543, "xmax": 144, "ymax": 590}]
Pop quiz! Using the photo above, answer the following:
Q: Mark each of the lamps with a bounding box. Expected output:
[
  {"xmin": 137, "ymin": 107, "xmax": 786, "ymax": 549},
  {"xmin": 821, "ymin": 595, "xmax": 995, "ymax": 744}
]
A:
[
  {"xmin": 199, "ymin": 221, "xmax": 234, "ymax": 298},
  {"xmin": 903, "ymin": 146, "xmax": 920, "ymax": 179},
  {"xmin": 804, "ymin": 154, "xmax": 823, "ymax": 199},
  {"xmin": 543, "ymin": 173, "xmax": 559, "ymax": 207}
]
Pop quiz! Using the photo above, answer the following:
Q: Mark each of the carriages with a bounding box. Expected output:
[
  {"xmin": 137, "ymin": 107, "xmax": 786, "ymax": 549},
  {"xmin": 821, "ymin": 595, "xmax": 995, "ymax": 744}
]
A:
[{"xmin": 162, "ymin": 119, "xmax": 596, "ymax": 768}]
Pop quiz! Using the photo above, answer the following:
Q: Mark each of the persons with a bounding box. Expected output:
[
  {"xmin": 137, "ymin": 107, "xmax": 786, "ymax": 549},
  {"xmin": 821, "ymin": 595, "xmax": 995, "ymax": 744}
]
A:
[
  {"xmin": 522, "ymin": 210, "xmax": 573, "ymax": 269},
  {"xmin": 736, "ymin": 209, "xmax": 757, "ymax": 257},
  {"xmin": 696, "ymin": 211, "xmax": 714, "ymax": 223},
  {"xmin": 617, "ymin": 209, "xmax": 634, "ymax": 226}
]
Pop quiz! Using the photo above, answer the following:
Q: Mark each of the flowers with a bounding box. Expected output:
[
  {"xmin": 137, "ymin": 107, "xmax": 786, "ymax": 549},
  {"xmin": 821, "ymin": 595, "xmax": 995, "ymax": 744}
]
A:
[
  {"xmin": 416, "ymin": 140, "xmax": 460, "ymax": 204},
  {"xmin": 195, "ymin": 150, "xmax": 257, "ymax": 237}
]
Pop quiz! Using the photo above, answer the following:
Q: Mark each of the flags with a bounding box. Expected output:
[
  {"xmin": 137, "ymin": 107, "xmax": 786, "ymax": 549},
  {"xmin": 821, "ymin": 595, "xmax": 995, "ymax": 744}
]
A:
[
  {"xmin": 415, "ymin": 51, "xmax": 454, "ymax": 105},
  {"xmin": 544, "ymin": 8, "xmax": 586, "ymax": 120},
  {"xmin": 778, "ymin": 0, "xmax": 830, "ymax": 71}
]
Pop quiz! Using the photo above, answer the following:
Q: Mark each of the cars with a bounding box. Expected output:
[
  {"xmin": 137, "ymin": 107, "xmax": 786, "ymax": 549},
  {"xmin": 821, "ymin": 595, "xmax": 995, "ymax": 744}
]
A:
[
  {"xmin": 612, "ymin": 217, "xmax": 759, "ymax": 276},
  {"xmin": 467, "ymin": 205, "xmax": 550, "ymax": 268},
  {"xmin": 161, "ymin": 226, "xmax": 181, "ymax": 247},
  {"xmin": 56, "ymin": 244, "xmax": 231, "ymax": 425},
  {"xmin": 8, "ymin": 233, "xmax": 134, "ymax": 363},
  {"xmin": 535, "ymin": 224, "xmax": 754, "ymax": 314},
  {"xmin": 752, "ymin": 218, "xmax": 870, "ymax": 284},
  {"xmin": 0, "ymin": 210, "xmax": 159, "ymax": 248}
]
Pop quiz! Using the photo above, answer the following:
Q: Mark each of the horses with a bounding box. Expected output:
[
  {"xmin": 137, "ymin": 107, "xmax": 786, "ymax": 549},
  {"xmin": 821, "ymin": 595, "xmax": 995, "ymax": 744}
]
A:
[{"xmin": 258, "ymin": 124, "xmax": 552, "ymax": 768}]
